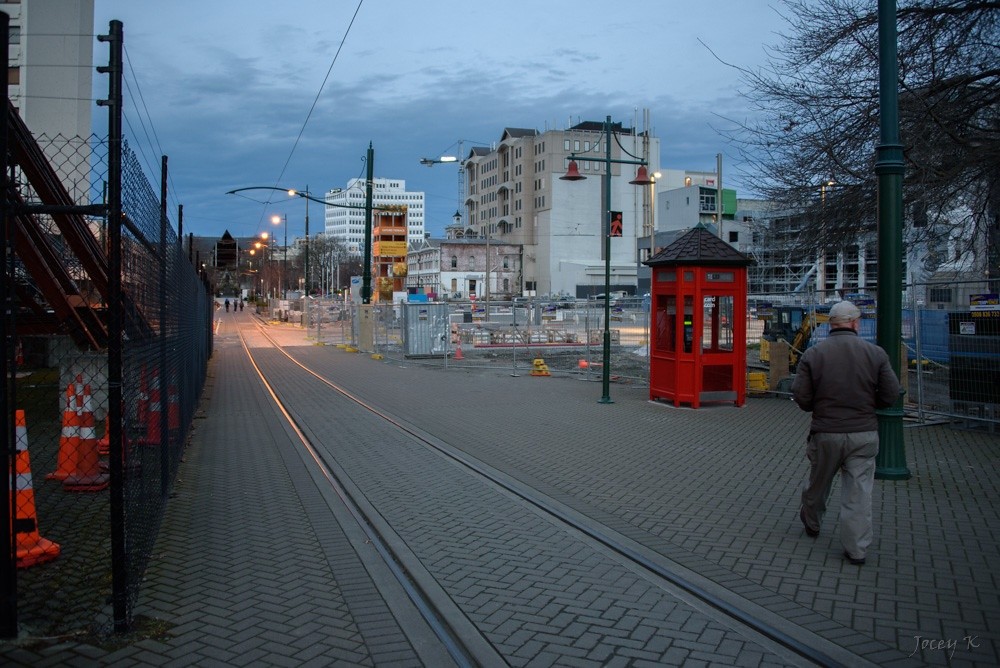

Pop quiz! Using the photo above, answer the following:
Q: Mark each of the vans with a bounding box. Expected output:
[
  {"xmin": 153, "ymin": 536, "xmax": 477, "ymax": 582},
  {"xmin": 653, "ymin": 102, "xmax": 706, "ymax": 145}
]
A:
[{"xmin": 845, "ymin": 293, "xmax": 876, "ymax": 317}]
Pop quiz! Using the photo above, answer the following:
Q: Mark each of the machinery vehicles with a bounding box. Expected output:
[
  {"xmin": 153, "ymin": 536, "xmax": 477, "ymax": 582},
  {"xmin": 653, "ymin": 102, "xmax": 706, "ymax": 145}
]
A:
[{"xmin": 759, "ymin": 301, "xmax": 837, "ymax": 372}]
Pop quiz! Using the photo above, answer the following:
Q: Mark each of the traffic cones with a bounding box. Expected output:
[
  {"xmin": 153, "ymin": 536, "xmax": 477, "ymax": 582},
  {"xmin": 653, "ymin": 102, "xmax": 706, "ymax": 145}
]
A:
[
  {"xmin": 61, "ymin": 383, "xmax": 111, "ymax": 493},
  {"xmin": 136, "ymin": 366, "xmax": 151, "ymax": 433},
  {"xmin": 43, "ymin": 384, "xmax": 79, "ymax": 480},
  {"xmin": 164, "ymin": 373, "xmax": 182, "ymax": 442},
  {"xmin": 6, "ymin": 410, "xmax": 61, "ymax": 569},
  {"xmin": 94, "ymin": 380, "xmax": 135, "ymax": 455},
  {"xmin": 74, "ymin": 373, "xmax": 87, "ymax": 417},
  {"xmin": 134, "ymin": 368, "xmax": 178, "ymax": 446},
  {"xmin": 15, "ymin": 340, "xmax": 25, "ymax": 370}
]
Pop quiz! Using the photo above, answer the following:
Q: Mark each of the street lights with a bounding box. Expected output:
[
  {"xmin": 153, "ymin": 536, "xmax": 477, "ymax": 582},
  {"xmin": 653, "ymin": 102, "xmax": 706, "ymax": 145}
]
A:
[
  {"xmin": 228, "ymin": 140, "xmax": 376, "ymax": 351},
  {"xmin": 563, "ymin": 114, "xmax": 658, "ymax": 406},
  {"xmin": 271, "ymin": 213, "xmax": 288, "ymax": 319},
  {"xmin": 254, "ymin": 242, "xmax": 266, "ymax": 300},
  {"xmin": 260, "ymin": 232, "xmax": 274, "ymax": 300},
  {"xmin": 290, "ymin": 186, "xmax": 316, "ymax": 325}
]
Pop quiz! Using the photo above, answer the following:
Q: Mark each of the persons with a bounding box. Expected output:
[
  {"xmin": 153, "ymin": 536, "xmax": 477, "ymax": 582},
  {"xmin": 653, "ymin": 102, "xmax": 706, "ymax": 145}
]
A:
[
  {"xmin": 225, "ymin": 299, "xmax": 230, "ymax": 312},
  {"xmin": 234, "ymin": 300, "xmax": 237, "ymax": 311},
  {"xmin": 791, "ymin": 300, "xmax": 899, "ymax": 565},
  {"xmin": 239, "ymin": 301, "xmax": 244, "ymax": 311}
]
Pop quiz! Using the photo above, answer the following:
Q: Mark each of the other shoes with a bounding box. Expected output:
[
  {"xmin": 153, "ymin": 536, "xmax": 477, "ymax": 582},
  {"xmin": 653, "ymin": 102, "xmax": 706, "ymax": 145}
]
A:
[
  {"xmin": 800, "ymin": 506, "xmax": 819, "ymax": 535},
  {"xmin": 844, "ymin": 549, "xmax": 865, "ymax": 564}
]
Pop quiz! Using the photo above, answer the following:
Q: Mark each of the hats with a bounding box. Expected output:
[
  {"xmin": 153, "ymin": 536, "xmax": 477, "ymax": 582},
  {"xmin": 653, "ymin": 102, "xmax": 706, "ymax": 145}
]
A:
[{"xmin": 829, "ymin": 300, "xmax": 861, "ymax": 323}]
